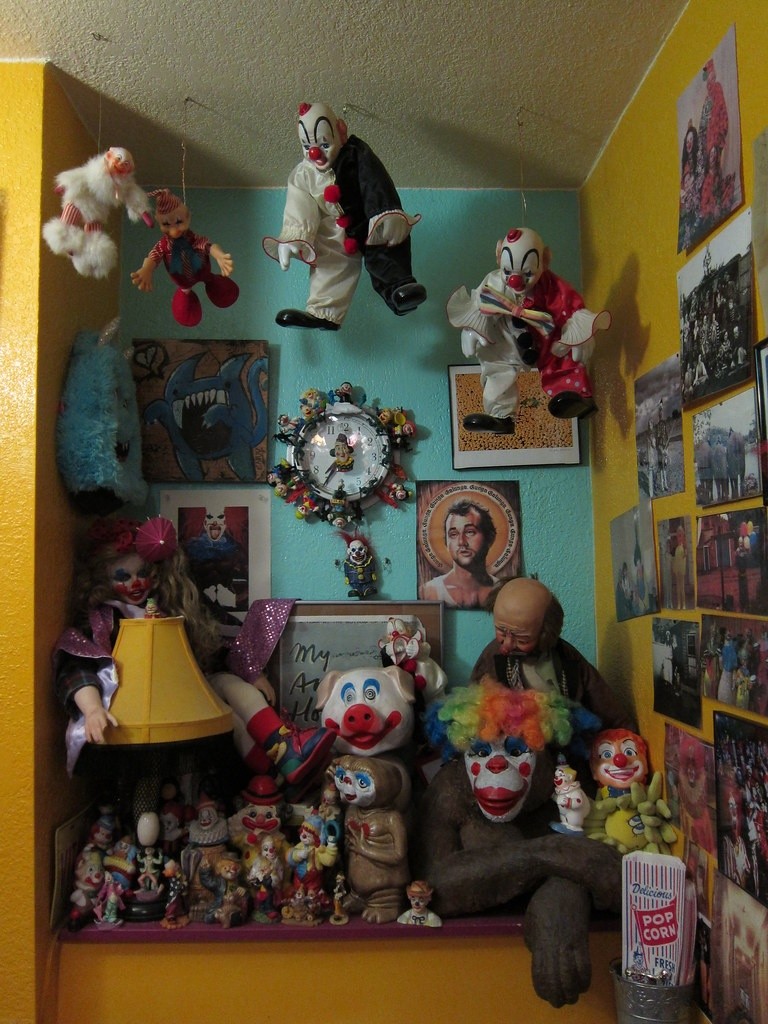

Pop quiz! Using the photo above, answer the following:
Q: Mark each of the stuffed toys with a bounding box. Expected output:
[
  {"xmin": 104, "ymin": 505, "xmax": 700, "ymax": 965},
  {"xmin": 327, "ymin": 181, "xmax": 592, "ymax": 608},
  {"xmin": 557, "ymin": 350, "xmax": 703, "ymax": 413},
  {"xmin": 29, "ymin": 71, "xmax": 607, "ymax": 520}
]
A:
[{"xmin": 55, "ymin": 331, "xmax": 146, "ymax": 514}]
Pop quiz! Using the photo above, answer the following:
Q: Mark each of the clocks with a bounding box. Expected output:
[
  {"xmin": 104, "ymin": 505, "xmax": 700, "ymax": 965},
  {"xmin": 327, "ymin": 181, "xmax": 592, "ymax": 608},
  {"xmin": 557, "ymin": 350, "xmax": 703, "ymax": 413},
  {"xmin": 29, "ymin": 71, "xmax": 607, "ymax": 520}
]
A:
[{"xmin": 291, "ymin": 405, "xmax": 390, "ymax": 503}]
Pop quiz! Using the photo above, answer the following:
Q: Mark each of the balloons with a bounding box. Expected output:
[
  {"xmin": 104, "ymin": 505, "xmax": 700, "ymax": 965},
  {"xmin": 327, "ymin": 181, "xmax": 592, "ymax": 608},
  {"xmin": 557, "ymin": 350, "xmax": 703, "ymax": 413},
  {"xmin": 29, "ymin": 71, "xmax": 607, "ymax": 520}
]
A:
[
  {"xmin": 739, "ymin": 522, "xmax": 747, "ymax": 536},
  {"xmin": 746, "ymin": 521, "xmax": 753, "ymax": 533},
  {"xmin": 744, "ymin": 536, "xmax": 750, "ymax": 549},
  {"xmin": 749, "ymin": 531, "xmax": 757, "ymax": 545}
]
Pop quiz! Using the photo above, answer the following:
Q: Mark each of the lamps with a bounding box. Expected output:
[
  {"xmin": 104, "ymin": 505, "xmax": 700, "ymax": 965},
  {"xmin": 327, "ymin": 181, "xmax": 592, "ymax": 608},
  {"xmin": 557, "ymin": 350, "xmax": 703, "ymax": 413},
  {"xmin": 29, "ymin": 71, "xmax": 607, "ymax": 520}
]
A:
[{"xmin": 94, "ymin": 615, "xmax": 234, "ymax": 921}]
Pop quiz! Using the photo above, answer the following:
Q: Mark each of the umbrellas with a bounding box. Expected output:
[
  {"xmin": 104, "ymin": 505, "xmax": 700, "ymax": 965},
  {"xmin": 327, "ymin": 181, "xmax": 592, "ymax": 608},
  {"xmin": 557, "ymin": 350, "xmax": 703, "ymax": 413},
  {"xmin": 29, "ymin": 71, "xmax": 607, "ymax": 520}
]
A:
[{"xmin": 135, "ymin": 515, "xmax": 179, "ymax": 561}]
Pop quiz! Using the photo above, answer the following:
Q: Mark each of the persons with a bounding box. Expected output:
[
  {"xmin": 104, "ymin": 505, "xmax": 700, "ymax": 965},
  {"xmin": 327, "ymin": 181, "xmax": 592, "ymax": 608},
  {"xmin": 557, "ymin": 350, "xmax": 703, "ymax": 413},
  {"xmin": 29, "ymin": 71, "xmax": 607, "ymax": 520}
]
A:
[
  {"xmin": 418, "ymin": 500, "xmax": 505, "ymax": 610},
  {"xmin": 43, "ymin": 98, "xmax": 612, "ymax": 431},
  {"xmin": 49, "ymin": 517, "xmax": 677, "ymax": 1008},
  {"xmin": 619, "ymin": 57, "xmax": 768, "ymax": 903}
]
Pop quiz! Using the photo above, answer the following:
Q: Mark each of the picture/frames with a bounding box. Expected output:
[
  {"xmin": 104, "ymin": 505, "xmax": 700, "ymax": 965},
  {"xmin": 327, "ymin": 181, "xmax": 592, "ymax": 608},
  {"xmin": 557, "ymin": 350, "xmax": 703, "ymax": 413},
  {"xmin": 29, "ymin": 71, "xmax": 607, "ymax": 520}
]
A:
[
  {"xmin": 264, "ymin": 600, "xmax": 446, "ymax": 728},
  {"xmin": 448, "ymin": 363, "xmax": 582, "ymax": 470},
  {"xmin": 158, "ymin": 490, "xmax": 270, "ymax": 636}
]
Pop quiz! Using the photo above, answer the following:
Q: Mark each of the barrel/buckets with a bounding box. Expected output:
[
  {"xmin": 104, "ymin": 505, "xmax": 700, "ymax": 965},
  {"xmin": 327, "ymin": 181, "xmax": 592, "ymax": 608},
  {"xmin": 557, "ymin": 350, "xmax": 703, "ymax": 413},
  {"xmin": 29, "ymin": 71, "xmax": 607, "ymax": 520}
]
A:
[{"xmin": 607, "ymin": 956, "xmax": 699, "ymax": 1024}]
